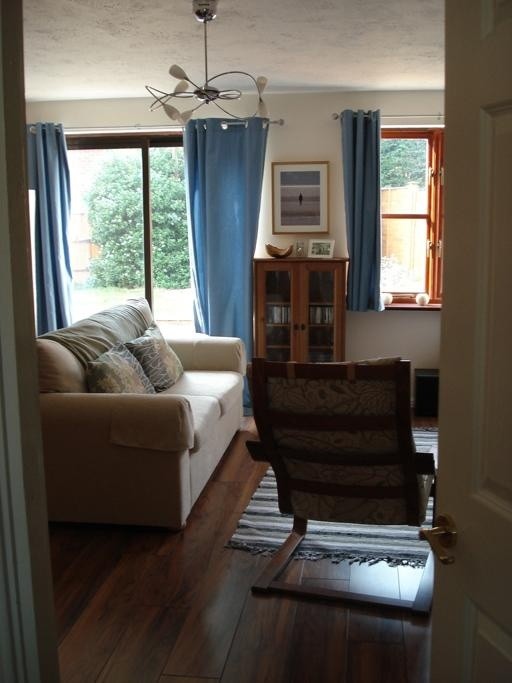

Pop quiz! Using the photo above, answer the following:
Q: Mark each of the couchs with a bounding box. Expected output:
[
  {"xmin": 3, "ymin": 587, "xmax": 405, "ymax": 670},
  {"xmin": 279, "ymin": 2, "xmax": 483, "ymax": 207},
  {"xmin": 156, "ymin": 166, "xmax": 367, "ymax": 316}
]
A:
[{"xmin": 34, "ymin": 296, "xmax": 247, "ymax": 533}]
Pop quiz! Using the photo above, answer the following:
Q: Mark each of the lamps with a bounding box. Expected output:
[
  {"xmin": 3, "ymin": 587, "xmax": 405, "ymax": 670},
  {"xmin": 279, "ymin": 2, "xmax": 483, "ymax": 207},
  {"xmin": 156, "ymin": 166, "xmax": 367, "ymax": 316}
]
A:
[{"xmin": 143, "ymin": 0, "xmax": 269, "ymax": 125}]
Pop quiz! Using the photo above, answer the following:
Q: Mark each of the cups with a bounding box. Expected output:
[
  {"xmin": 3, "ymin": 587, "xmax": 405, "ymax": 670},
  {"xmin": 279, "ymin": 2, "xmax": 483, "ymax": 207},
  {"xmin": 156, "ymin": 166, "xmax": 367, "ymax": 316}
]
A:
[{"xmin": 295, "ymin": 240, "xmax": 305, "ymax": 256}]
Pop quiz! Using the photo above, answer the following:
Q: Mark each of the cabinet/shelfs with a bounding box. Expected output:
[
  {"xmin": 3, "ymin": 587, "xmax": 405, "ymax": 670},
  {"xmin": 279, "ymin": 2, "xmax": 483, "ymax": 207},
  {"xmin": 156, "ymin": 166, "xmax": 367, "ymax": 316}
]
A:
[{"xmin": 251, "ymin": 257, "xmax": 349, "ymax": 363}]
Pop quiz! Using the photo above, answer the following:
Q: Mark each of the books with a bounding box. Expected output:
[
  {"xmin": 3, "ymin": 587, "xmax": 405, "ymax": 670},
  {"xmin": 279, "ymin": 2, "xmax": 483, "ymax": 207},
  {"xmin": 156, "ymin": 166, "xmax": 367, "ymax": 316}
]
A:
[{"xmin": 266, "ymin": 305, "xmax": 334, "ymax": 325}]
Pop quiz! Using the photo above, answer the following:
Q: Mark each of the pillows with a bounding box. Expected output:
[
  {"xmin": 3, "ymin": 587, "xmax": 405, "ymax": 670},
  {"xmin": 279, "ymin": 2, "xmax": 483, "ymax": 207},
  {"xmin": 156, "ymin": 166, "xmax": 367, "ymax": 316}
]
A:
[
  {"xmin": 84, "ymin": 339, "xmax": 156, "ymax": 394},
  {"xmin": 123, "ymin": 321, "xmax": 185, "ymax": 394}
]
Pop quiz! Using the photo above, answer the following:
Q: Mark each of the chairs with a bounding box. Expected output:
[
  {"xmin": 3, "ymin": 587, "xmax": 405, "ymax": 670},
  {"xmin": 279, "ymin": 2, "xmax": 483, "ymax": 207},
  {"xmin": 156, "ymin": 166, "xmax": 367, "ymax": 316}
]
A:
[{"xmin": 243, "ymin": 356, "xmax": 436, "ymax": 622}]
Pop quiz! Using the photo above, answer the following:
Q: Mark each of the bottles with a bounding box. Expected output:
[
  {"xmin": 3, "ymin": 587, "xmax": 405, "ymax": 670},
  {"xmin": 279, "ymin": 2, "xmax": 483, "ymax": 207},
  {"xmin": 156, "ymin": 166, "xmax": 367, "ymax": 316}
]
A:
[
  {"xmin": 415, "ymin": 293, "xmax": 430, "ymax": 306},
  {"xmin": 381, "ymin": 293, "xmax": 393, "ymax": 305}
]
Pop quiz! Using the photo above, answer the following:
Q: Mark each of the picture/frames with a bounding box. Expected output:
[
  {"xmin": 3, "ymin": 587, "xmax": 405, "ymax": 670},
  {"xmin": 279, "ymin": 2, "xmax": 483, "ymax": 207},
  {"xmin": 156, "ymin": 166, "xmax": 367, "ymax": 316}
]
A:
[
  {"xmin": 270, "ymin": 161, "xmax": 330, "ymax": 235},
  {"xmin": 307, "ymin": 238, "xmax": 336, "ymax": 259}
]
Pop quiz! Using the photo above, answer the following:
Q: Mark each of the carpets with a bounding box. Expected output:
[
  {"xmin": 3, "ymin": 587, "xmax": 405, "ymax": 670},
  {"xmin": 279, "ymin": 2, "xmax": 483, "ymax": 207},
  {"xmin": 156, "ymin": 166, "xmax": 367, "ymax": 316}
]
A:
[{"xmin": 221, "ymin": 424, "xmax": 438, "ymax": 569}]
